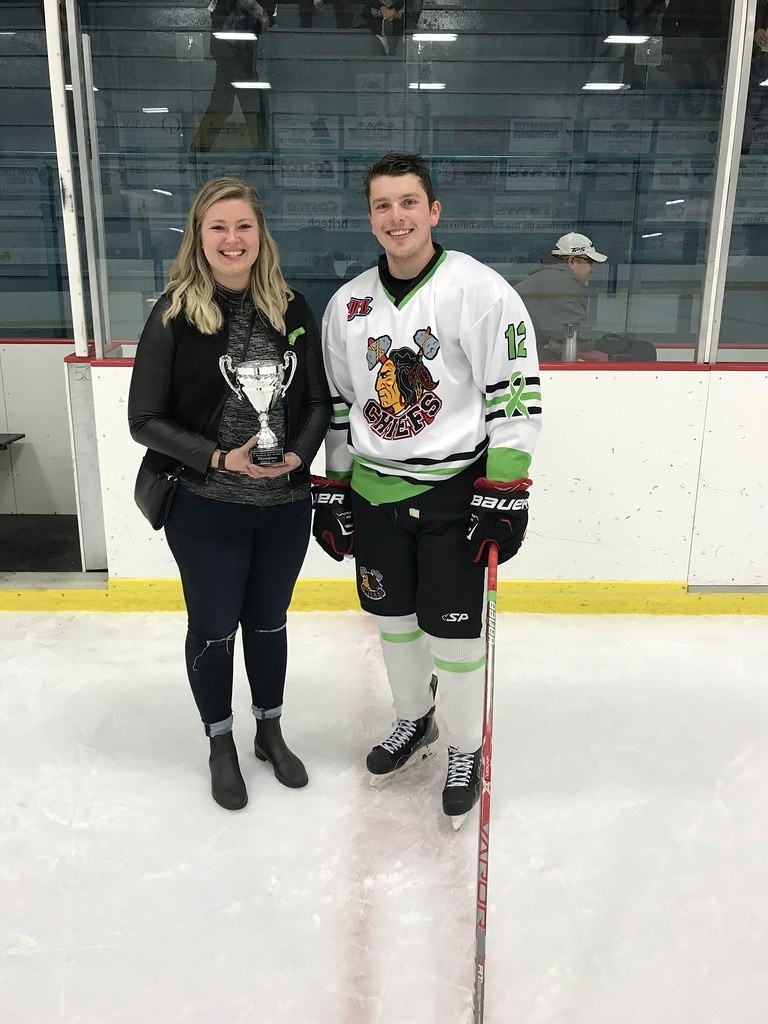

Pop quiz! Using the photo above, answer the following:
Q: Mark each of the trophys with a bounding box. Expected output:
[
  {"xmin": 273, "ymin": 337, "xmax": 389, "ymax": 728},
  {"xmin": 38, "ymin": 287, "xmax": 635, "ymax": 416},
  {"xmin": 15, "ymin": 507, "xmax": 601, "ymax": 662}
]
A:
[{"xmin": 219, "ymin": 351, "xmax": 297, "ymax": 466}]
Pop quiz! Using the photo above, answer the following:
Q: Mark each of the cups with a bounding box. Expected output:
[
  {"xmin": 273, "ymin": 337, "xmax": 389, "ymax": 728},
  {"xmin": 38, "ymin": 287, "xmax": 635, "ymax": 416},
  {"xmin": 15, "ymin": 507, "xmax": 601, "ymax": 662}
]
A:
[{"xmin": 562, "ymin": 324, "xmax": 581, "ymax": 361}]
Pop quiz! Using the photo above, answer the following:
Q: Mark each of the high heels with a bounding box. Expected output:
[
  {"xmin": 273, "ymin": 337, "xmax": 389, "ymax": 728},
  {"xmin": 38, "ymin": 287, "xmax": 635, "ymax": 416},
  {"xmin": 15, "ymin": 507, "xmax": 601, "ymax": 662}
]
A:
[
  {"xmin": 209, "ymin": 729, "xmax": 248, "ymax": 810},
  {"xmin": 254, "ymin": 716, "xmax": 309, "ymax": 788}
]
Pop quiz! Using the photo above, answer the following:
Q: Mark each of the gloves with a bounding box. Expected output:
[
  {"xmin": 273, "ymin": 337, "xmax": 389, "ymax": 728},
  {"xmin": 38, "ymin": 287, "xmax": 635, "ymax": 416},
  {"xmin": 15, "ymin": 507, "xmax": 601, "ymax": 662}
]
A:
[
  {"xmin": 309, "ymin": 474, "xmax": 354, "ymax": 562},
  {"xmin": 457, "ymin": 477, "xmax": 532, "ymax": 568}
]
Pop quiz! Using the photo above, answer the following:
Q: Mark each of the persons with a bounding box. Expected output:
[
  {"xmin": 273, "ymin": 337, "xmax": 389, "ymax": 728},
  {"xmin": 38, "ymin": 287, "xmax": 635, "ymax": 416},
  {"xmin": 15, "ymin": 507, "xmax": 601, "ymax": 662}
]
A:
[
  {"xmin": 513, "ymin": 233, "xmax": 608, "ymax": 365},
  {"xmin": 298, "ymin": 0, "xmax": 423, "ymax": 57},
  {"xmin": 617, "ymin": 0, "xmax": 768, "ymax": 176},
  {"xmin": 129, "ymin": 178, "xmax": 334, "ymax": 811},
  {"xmin": 189, "ymin": 0, "xmax": 283, "ymax": 164},
  {"xmin": 313, "ymin": 155, "xmax": 542, "ymax": 829}
]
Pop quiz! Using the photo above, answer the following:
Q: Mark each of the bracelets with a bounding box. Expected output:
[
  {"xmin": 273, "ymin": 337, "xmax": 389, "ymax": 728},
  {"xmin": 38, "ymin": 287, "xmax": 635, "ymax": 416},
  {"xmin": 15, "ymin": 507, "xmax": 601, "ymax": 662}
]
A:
[{"xmin": 218, "ymin": 450, "xmax": 239, "ymax": 478}]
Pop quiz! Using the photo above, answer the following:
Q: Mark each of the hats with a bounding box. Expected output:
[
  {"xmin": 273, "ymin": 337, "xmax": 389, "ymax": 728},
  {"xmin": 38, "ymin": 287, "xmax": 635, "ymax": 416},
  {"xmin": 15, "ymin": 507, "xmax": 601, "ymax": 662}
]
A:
[{"xmin": 551, "ymin": 231, "xmax": 607, "ymax": 262}]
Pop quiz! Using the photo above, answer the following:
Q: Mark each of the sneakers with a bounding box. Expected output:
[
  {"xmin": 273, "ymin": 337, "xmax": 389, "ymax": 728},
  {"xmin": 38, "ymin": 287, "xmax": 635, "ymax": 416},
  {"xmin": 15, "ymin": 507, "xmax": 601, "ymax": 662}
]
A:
[
  {"xmin": 366, "ymin": 673, "xmax": 439, "ymax": 791},
  {"xmin": 376, "ymin": 34, "xmax": 390, "ymax": 56},
  {"xmin": 442, "ymin": 745, "xmax": 484, "ymax": 831}
]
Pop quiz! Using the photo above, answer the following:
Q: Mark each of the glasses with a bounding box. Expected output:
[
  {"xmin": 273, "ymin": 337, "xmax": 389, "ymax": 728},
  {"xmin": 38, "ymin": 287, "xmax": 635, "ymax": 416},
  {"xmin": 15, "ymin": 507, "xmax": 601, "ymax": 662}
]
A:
[{"xmin": 567, "ymin": 256, "xmax": 594, "ymax": 264}]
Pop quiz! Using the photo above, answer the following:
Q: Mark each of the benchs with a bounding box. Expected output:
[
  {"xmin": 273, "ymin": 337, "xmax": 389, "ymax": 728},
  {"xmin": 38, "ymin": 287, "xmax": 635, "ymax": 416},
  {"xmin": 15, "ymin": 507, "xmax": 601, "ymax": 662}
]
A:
[{"xmin": 0, "ymin": 0, "xmax": 768, "ymax": 343}]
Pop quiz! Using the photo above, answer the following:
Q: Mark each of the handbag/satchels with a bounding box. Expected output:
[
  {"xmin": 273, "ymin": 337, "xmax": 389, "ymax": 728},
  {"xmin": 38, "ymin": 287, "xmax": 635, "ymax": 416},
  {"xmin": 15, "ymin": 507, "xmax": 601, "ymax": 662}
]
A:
[
  {"xmin": 134, "ymin": 456, "xmax": 185, "ymax": 530},
  {"xmin": 371, "ymin": 0, "xmax": 405, "ymax": 20}
]
